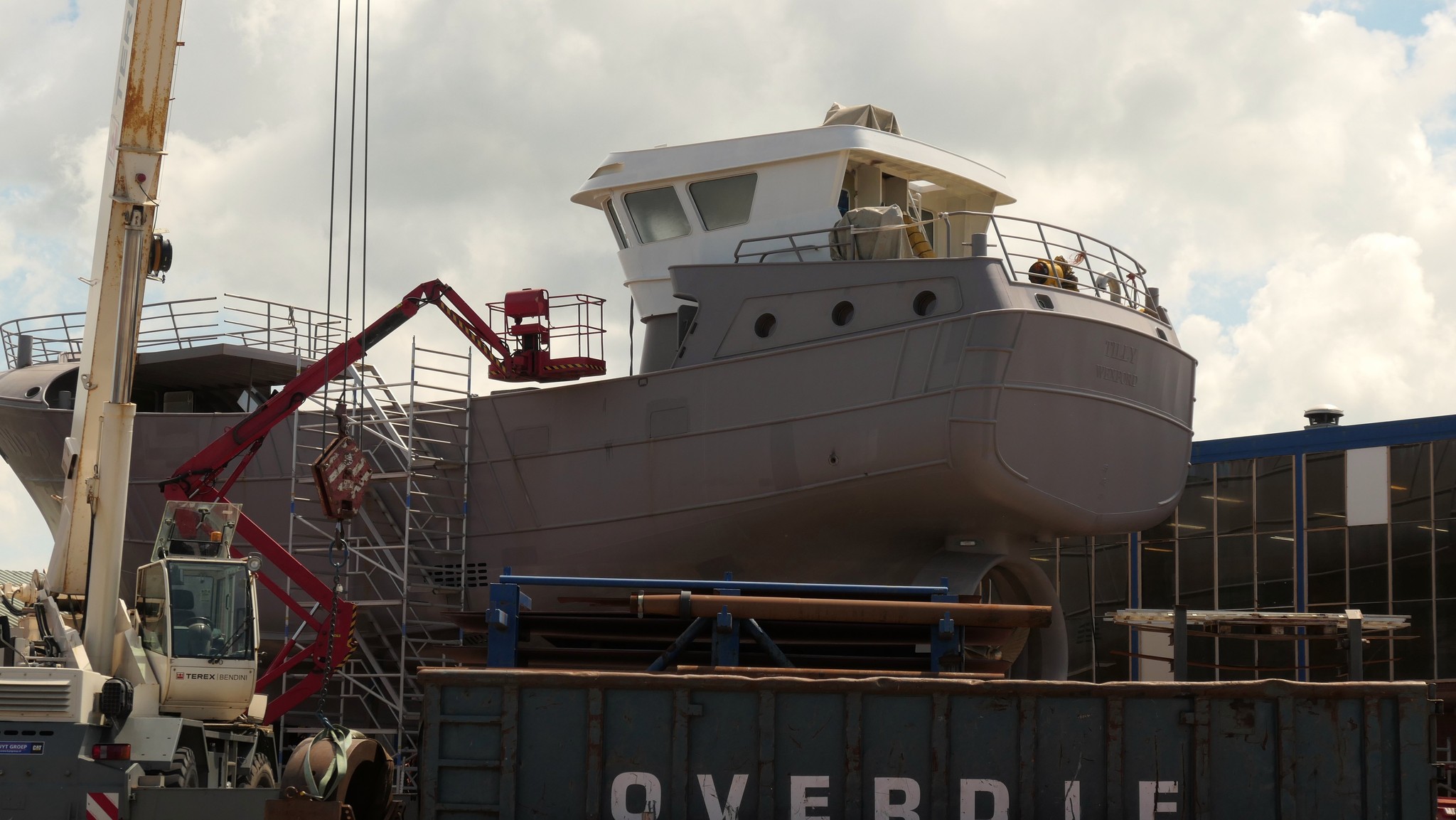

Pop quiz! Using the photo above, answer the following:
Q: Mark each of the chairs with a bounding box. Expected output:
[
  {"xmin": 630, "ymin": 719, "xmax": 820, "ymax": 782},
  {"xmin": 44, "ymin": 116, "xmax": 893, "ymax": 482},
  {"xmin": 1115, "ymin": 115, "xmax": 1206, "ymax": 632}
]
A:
[{"xmin": 171, "ymin": 590, "xmax": 198, "ymax": 627}]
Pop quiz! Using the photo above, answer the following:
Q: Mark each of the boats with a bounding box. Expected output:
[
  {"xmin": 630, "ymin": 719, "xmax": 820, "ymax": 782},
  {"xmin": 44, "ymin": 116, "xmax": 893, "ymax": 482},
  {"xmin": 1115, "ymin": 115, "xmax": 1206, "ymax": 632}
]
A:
[{"xmin": 1, "ymin": 102, "xmax": 1204, "ymax": 671}]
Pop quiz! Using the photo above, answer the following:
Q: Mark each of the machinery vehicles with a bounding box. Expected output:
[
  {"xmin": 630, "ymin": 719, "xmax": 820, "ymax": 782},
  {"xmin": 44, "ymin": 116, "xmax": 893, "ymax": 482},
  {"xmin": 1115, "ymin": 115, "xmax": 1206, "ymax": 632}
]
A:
[{"xmin": 0, "ymin": 0, "xmax": 612, "ymax": 820}]
